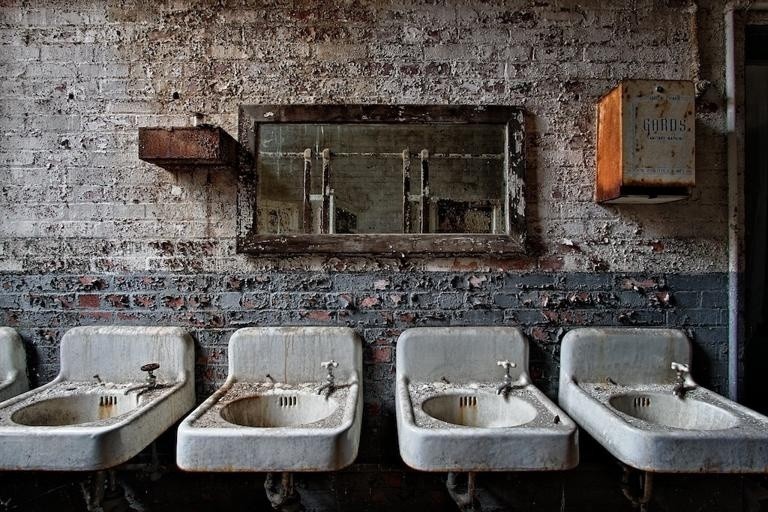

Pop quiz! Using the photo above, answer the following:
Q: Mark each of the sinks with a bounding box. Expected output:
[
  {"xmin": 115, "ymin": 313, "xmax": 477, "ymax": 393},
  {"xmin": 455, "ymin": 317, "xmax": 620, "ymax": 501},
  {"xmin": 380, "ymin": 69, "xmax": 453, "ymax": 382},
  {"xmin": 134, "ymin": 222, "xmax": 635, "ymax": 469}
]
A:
[
  {"xmin": 177, "ymin": 326, "xmax": 363, "ymax": 471},
  {"xmin": 396, "ymin": 329, "xmax": 578, "ymax": 471},
  {"xmin": 0, "ymin": 326, "xmax": 195, "ymax": 473},
  {"xmin": 0, "ymin": 326, "xmax": 30, "ymax": 403},
  {"xmin": 560, "ymin": 326, "xmax": 768, "ymax": 474}
]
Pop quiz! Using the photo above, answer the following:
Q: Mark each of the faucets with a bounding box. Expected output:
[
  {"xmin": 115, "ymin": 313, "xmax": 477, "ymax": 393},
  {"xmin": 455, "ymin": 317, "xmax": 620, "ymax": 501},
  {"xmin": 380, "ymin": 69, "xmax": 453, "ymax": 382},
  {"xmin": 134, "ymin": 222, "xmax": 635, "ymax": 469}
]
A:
[
  {"xmin": 672, "ymin": 360, "xmax": 692, "ymax": 397},
  {"xmin": 125, "ymin": 363, "xmax": 162, "ymax": 397},
  {"xmin": 496, "ymin": 358, "xmax": 515, "ymax": 397},
  {"xmin": 317, "ymin": 358, "xmax": 339, "ymax": 396}
]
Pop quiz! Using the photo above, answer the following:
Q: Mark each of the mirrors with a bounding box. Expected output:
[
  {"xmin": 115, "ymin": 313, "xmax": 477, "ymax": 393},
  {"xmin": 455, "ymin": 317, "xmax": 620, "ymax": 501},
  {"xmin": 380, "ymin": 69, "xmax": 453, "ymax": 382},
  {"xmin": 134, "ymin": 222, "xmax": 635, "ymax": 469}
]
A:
[{"xmin": 236, "ymin": 103, "xmax": 531, "ymax": 259}]
